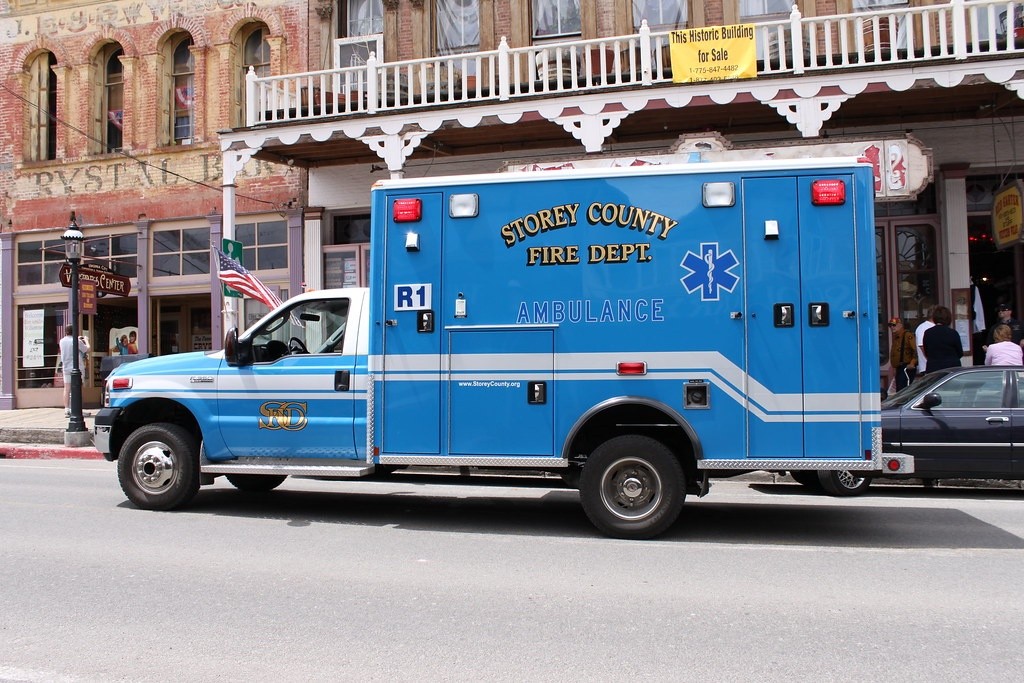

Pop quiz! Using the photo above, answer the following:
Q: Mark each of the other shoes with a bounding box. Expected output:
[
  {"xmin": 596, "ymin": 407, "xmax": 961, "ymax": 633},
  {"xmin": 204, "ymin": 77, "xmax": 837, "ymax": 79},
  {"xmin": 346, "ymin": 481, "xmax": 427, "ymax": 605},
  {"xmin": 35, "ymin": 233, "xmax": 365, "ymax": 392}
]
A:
[
  {"xmin": 82, "ymin": 410, "xmax": 91, "ymax": 417},
  {"xmin": 64, "ymin": 411, "xmax": 71, "ymax": 418}
]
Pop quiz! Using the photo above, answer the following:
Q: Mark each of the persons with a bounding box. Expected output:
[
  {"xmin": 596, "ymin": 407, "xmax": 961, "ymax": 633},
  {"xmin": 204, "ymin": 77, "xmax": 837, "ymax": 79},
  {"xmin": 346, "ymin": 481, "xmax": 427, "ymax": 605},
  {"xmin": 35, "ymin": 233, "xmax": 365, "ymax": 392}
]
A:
[
  {"xmin": 887, "ymin": 317, "xmax": 919, "ymax": 393},
  {"xmin": 982, "ymin": 325, "xmax": 1024, "ymax": 366},
  {"xmin": 915, "ymin": 307, "xmax": 937, "ymax": 376},
  {"xmin": 60, "ymin": 323, "xmax": 91, "ymax": 418},
  {"xmin": 987, "ymin": 303, "xmax": 1024, "ymax": 347},
  {"xmin": 923, "ymin": 306, "xmax": 964, "ymax": 374}
]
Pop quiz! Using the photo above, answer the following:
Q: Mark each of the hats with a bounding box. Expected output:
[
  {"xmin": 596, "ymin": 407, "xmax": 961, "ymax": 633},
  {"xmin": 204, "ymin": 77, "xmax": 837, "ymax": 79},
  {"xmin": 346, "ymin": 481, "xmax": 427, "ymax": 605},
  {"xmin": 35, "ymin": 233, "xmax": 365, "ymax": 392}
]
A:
[
  {"xmin": 996, "ymin": 294, "xmax": 1017, "ymax": 306},
  {"xmin": 888, "ymin": 318, "xmax": 901, "ymax": 325}
]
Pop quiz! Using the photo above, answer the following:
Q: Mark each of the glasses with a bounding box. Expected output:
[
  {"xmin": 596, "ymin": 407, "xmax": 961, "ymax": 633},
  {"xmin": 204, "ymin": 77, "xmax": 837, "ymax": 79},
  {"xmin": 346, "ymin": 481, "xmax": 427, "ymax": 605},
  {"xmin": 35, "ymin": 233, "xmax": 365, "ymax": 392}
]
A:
[
  {"xmin": 994, "ymin": 305, "xmax": 1010, "ymax": 312},
  {"xmin": 888, "ymin": 324, "xmax": 896, "ymax": 327}
]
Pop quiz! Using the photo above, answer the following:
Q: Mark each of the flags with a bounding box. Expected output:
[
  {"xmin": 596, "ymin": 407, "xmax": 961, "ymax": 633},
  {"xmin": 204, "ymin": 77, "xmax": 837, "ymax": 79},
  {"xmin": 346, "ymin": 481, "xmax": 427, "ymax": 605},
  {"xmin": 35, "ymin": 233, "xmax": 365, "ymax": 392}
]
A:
[{"xmin": 213, "ymin": 246, "xmax": 304, "ymax": 329}]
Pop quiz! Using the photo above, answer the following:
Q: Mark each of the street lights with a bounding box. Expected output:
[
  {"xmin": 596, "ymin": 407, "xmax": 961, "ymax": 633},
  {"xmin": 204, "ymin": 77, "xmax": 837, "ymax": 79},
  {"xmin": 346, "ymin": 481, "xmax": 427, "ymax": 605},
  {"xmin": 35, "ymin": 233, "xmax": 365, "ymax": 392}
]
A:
[{"xmin": 59, "ymin": 212, "xmax": 93, "ymax": 449}]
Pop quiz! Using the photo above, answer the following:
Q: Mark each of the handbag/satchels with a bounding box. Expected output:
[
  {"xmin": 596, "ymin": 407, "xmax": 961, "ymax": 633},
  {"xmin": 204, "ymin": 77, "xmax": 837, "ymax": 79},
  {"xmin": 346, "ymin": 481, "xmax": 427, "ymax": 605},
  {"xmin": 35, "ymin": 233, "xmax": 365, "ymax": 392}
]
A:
[{"xmin": 895, "ymin": 364, "xmax": 916, "ymax": 392}]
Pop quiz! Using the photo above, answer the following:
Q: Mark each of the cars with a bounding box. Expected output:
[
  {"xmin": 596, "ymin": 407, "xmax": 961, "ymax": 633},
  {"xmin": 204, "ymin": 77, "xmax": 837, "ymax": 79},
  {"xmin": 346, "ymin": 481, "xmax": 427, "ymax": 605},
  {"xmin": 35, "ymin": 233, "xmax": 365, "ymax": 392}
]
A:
[{"xmin": 789, "ymin": 366, "xmax": 1024, "ymax": 497}]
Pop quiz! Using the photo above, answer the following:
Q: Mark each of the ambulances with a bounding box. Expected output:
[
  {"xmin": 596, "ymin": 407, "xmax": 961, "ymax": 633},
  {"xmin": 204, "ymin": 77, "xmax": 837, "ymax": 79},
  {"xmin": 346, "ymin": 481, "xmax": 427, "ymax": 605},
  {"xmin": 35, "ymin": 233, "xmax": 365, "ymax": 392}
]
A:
[{"xmin": 89, "ymin": 157, "xmax": 883, "ymax": 541}]
